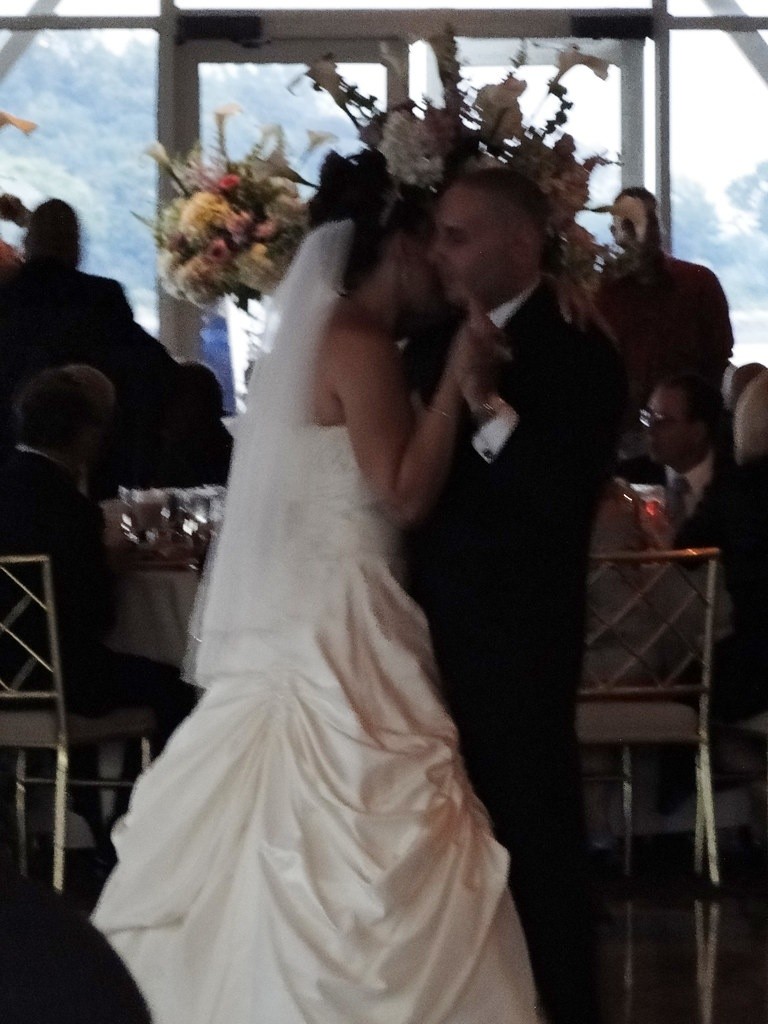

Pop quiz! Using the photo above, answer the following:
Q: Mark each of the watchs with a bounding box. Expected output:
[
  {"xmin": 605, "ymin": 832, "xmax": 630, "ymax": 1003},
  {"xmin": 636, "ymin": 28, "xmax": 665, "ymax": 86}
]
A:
[{"xmin": 472, "ymin": 393, "xmax": 505, "ymax": 420}]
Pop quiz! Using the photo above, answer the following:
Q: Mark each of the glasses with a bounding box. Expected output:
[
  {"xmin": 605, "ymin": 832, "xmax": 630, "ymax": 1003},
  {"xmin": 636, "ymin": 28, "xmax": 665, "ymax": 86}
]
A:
[{"xmin": 638, "ymin": 407, "xmax": 676, "ymax": 431}]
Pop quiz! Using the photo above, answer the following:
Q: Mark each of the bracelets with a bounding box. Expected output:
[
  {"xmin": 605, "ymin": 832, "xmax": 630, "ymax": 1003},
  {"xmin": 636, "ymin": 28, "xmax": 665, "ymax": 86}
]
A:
[{"xmin": 426, "ymin": 404, "xmax": 459, "ymax": 422}]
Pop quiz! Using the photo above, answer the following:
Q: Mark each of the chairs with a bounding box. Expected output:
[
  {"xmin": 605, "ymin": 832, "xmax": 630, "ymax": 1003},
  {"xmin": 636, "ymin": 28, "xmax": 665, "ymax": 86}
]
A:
[
  {"xmin": 573, "ymin": 548, "xmax": 722, "ymax": 889},
  {"xmin": 0, "ymin": 554, "xmax": 154, "ymax": 894}
]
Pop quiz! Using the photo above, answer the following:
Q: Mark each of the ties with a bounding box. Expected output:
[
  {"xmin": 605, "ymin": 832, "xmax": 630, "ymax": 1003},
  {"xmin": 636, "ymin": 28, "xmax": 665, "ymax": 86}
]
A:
[{"xmin": 664, "ymin": 477, "xmax": 692, "ymax": 530}]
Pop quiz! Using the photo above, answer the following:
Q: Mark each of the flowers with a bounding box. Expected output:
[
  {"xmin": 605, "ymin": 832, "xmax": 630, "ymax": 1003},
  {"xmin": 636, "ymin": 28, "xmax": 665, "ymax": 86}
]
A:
[
  {"xmin": 128, "ymin": 104, "xmax": 340, "ymax": 321},
  {"xmin": 305, "ymin": 20, "xmax": 632, "ymax": 352}
]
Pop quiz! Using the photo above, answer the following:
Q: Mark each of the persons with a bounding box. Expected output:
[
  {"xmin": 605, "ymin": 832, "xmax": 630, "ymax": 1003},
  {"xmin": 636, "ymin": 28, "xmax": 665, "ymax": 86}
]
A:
[
  {"xmin": 1, "ymin": 191, "xmax": 236, "ymax": 500},
  {"xmin": 87, "ymin": 137, "xmax": 611, "ymax": 1024},
  {"xmin": 593, "ymin": 184, "xmax": 768, "ymax": 853},
  {"xmin": 1, "ymin": 366, "xmax": 201, "ymax": 871},
  {"xmin": 400, "ymin": 165, "xmax": 629, "ymax": 1024}
]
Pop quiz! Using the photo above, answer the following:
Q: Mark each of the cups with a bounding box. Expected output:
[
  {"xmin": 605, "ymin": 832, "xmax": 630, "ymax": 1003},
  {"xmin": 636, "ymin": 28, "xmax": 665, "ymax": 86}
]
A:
[{"xmin": 118, "ymin": 485, "xmax": 224, "ymax": 564}]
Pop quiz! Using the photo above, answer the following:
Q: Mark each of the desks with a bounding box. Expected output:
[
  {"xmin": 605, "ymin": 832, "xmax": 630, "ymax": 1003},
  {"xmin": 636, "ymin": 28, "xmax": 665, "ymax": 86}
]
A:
[{"xmin": 110, "ymin": 570, "xmax": 751, "ymax": 846}]
